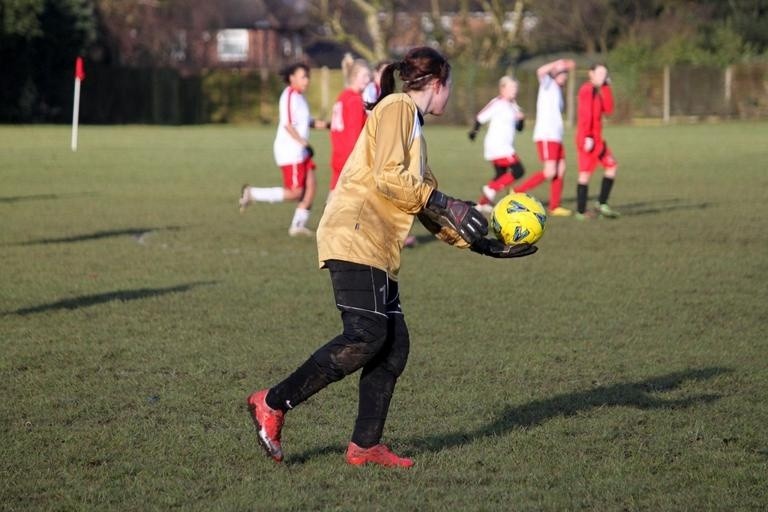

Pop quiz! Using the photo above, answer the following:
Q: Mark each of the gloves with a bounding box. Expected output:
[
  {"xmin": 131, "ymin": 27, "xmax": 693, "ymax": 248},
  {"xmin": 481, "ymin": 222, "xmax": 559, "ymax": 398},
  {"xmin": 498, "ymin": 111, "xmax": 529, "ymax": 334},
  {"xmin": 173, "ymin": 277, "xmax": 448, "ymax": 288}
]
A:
[
  {"xmin": 468, "ymin": 237, "xmax": 536, "ymax": 257},
  {"xmin": 425, "ymin": 191, "xmax": 488, "ymax": 243}
]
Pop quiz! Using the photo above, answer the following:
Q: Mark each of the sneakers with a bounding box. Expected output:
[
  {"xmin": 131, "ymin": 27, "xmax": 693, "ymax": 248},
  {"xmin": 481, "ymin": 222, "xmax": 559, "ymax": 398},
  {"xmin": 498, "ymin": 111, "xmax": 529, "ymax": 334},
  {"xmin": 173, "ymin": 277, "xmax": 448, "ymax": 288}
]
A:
[
  {"xmin": 346, "ymin": 441, "xmax": 415, "ymax": 468},
  {"xmin": 475, "ymin": 203, "xmax": 494, "ymax": 213},
  {"xmin": 549, "ymin": 202, "xmax": 621, "ymax": 219},
  {"xmin": 483, "ymin": 186, "xmax": 496, "ymax": 202},
  {"xmin": 239, "ymin": 184, "xmax": 252, "ymax": 214},
  {"xmin": 247, "ymin": 388, "xmax": 283, "ymax": 462},
  {"xmin": 289, "ymin": 226, "xmax": 315, "ymax": 237}
]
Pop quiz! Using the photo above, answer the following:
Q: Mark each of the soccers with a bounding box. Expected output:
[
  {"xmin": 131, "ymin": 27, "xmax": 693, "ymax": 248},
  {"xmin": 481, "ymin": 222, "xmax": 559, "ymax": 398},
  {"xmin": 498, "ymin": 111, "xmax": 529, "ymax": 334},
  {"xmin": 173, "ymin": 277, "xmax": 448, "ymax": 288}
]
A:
[{"xmin": 490, "ymin": 191, "xmax": 548, "ymax": 247}]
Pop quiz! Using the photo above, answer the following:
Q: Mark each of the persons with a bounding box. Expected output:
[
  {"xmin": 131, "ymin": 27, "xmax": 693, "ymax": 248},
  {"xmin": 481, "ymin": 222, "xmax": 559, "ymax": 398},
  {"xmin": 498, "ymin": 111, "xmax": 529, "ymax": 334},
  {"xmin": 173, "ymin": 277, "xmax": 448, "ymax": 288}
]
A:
[
  {"xmin": 245, "ymin": 46, "xmax": 537, "ymax": 467},
  {"xmin": 475, "ymin": 75, "xmax": 526, "ymax": 213},
  {"xmin": 575, "ymin": 63, "xmax": 620, "ymax": 221},
  {"xmin": 239, "ymin": 63, "xmax": 330, "ymax": 236},
  {"xmin": 361, "ymin": 61, "xmax": 392, "ymax": 114},
  {"xmin": 330, "ymin": 52, "xmax": 416, "ymax": 247},
  {"xmin": 508, "ymin": 59, "xmax": 575, "ymax": 217}
]
